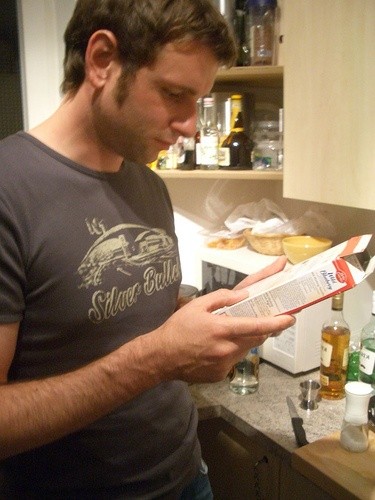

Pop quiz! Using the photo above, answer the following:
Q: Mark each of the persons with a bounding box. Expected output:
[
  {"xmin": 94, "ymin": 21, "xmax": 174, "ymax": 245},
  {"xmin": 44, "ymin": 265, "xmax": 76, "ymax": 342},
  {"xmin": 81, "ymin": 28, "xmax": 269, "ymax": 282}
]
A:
[{"xmin": 0, "ymin": 0, "xmax": 296, "ymax": 500}]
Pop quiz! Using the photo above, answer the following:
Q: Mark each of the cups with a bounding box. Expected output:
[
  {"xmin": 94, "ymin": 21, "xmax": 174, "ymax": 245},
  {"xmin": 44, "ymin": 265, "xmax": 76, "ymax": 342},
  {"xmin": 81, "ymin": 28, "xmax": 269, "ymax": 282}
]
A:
[
  {"xmin": 300, "ymin": 379, "xmax": 321, "ymax": 410},
  {"xmin": 229, "ymin": 344, "xmax": 261, "ymax": 395},
  {"xmin": 209, "ymin": 0, "xmax": 277, "ymax": 66}
]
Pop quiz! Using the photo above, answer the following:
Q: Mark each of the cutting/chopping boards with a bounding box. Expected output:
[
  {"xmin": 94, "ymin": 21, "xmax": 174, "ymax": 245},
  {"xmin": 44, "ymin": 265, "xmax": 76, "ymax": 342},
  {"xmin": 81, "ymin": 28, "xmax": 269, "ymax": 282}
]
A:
[{"xmin": 291, "ymin": 421, "xmax": 375, "ymax": 500}]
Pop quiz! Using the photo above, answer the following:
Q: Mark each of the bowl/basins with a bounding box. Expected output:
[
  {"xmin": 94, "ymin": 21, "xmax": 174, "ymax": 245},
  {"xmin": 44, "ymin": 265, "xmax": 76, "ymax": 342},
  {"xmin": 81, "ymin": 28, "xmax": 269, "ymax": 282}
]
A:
[
  {"xmin": 243, "ymin": 228, "xmax": 305, "ymax": 255},
  {"xmin": 282, "ymin": 235, "xmax": 332, "ymax": 265}
]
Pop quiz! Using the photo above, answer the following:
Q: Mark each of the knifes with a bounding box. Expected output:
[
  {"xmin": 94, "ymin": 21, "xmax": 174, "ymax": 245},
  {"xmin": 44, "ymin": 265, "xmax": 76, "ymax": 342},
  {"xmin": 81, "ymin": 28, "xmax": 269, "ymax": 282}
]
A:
[{"xmin": 286, "ymin": 394, "xmax": 308, "ymax": 447}]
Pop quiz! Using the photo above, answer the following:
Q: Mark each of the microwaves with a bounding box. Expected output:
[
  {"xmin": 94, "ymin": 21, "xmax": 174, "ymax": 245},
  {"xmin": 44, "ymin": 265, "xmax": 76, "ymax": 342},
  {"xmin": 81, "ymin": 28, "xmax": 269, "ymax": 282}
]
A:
[{"xmin": 192, "ymin": 235, "xmax": 375, "ymax": 374}]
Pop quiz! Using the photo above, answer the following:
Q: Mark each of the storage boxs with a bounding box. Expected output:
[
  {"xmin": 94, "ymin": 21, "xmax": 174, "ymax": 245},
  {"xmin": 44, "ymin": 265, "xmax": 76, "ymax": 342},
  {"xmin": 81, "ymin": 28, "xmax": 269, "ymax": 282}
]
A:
[{"xmin": 209, "ymin": 232, "xmax": 375, "ymax": 319}]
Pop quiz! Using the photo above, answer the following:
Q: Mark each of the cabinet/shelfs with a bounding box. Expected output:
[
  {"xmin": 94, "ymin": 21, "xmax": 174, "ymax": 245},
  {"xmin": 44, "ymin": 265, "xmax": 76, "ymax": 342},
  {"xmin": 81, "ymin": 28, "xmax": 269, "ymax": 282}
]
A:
[
  {"xmin": 201, "ymin": 418, "xmax": 328, "ymax": 500},
  {"xmin": 147, "ymin": 0, "xmax": 286, "ymax": 180}
]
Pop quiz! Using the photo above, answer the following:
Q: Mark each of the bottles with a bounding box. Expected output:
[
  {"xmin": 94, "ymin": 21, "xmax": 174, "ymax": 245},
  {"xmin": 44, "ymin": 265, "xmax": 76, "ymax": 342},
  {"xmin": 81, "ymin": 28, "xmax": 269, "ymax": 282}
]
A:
[
  {"xmin": 318, "ymin": 291, "xmax": 351, "ymax": 400},
  {"xmin": 340, "ymin": 381, "xmax": 374, "ymax": 452},
  {"xmin": 146, "ymin": 91, "xmax": 281, "ymax": 171},
  {"xmin": 358, "ymin": 289, "xmax": 375, "ymax": 423}
]
